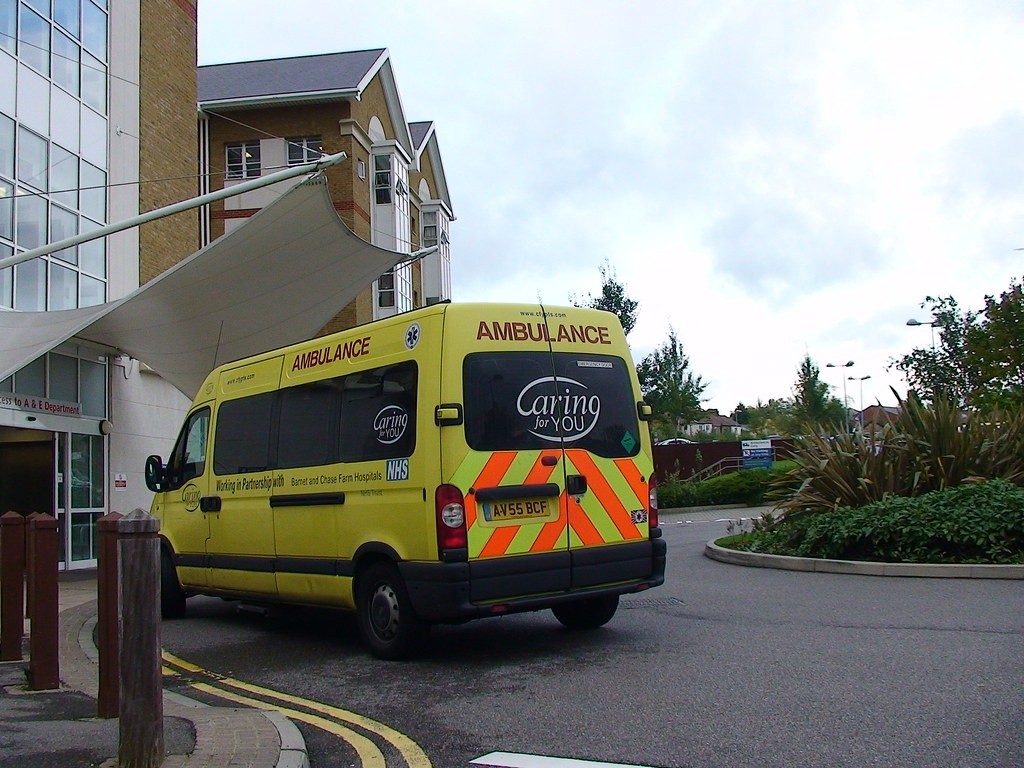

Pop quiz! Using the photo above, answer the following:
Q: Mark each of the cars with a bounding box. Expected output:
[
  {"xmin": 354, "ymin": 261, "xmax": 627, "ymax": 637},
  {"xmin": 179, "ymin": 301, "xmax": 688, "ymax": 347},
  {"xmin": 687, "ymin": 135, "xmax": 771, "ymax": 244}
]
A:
[
  {"xmin": 768, "ymin": 431, "xmax": 883, "ymax": 457},
  {"xmin": 655, "ymin": 438, "xmax": 691, "ymax": 445}
]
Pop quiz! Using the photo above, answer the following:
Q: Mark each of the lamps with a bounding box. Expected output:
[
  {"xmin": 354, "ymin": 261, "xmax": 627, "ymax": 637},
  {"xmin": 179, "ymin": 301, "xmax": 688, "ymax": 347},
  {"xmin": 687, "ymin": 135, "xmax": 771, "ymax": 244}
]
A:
[{"xmin": 99, "ymin": 419, "xmax": 114, "ymax": 435}]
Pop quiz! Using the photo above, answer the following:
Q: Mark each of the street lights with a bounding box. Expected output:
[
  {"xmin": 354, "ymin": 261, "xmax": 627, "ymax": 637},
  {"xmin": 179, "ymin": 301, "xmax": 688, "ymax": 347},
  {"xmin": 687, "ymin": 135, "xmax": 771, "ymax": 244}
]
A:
[
  {"xmin": 735, "ymin": 410, "xmax": 742, "ymax": 440},
  {"xmin": 848, "ymin": 376, "xmax": 871, "ymax": 431},
  {"xmin": 826, "ymin": 361, "xmax": 855, "ymax": 435},
  {"xmin": 906, "ymin": 319, "xmax": 936, "ymax": 399}
]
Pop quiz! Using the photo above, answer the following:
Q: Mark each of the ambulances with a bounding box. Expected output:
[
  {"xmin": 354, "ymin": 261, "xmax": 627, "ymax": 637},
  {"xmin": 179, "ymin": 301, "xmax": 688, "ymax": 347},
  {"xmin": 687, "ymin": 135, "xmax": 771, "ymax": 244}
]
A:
[{"xmin": 145, "ymin": 299, "xmax": 666, "ymax": 662}]
